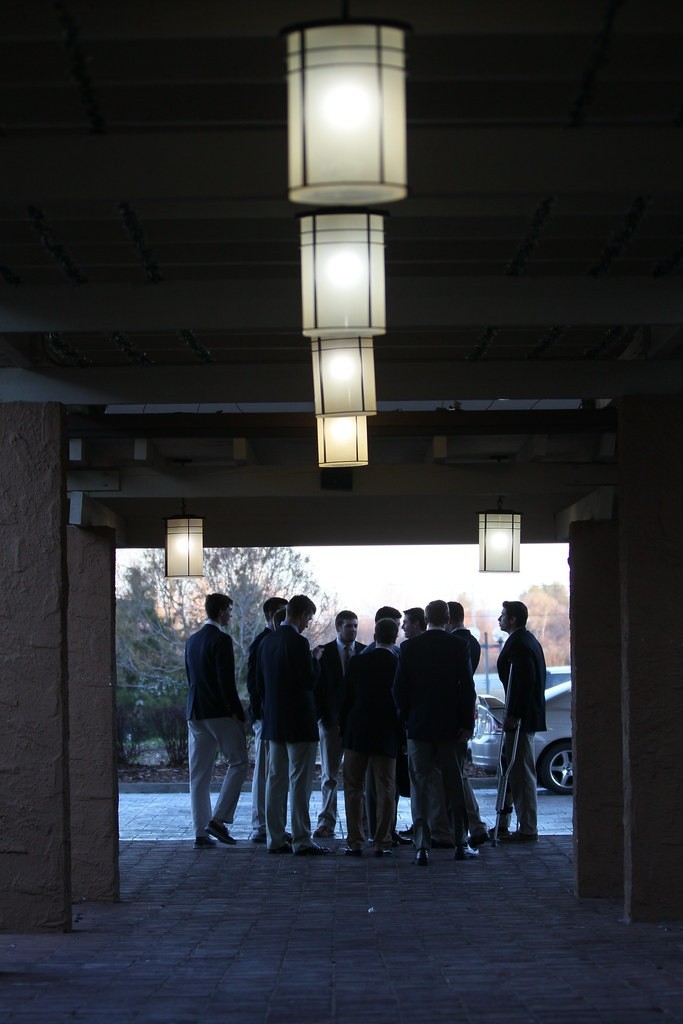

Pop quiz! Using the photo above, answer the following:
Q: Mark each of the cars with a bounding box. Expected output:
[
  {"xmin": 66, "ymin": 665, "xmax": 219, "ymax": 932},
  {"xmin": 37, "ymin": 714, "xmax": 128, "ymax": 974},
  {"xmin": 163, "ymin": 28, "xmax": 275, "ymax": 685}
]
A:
[{"xmin": 471, "ymin": 679, "xmax": 573, "ymax": 794}]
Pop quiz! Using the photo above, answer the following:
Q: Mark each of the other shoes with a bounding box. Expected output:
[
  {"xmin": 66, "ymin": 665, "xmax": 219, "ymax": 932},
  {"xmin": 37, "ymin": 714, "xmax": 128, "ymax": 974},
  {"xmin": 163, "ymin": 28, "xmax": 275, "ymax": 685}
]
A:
[
  {"xmin": 509, "ymin": 830, "xmax": 539, "ymax": 843},
  {"xmin": 252, "ymin": 832, "xmax": 291, "ymax": 843},
  {"xmin": 397, "ymin": 834, "xmax": 414, "ymax": 845},
  {"xmin": 374, "ymin": 850, "xmax": 392, "ymax": 858},
  {"xmin": 399, "ymin": 824, "xmax": 413, "ymax": 836},
  {"xmin": 413, "ymin": 838, "xmax": 448, "ymax": 849},
  {"xmin": 344, "ymin": 845, "xmax": 357, "ymax": 856},
  {"xmin": 313, "ymin": 826, "xmax": 334, "ymax": 839},
  {"xmin": 488, "ymin": 826, "xmax": 508, "ymax": 834},
  {"xmin": 267, "ymin": 842, "xmax": 293, "ymax": 853},
  {"xmin": 293, "ymin": 842, "xmax": 331, "ymax": 856},
  {"xmin": 391, "ymin": 840, "xmax": 399, "ymax": 847}
]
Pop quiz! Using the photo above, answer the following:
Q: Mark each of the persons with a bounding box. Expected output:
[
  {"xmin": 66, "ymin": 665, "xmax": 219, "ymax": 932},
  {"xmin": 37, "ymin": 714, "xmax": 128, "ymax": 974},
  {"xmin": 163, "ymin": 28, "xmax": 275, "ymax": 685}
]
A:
[
  {"xmin": 184, "ymin": 593, "xmax": 249, "ymax": 849},
  {"xmin": 247, "ymin": 595, "xmax": 491, "ymax": 866},
  {"xmin": 488, "ymin": 600, "xmax": 547, "ymax": 846}
]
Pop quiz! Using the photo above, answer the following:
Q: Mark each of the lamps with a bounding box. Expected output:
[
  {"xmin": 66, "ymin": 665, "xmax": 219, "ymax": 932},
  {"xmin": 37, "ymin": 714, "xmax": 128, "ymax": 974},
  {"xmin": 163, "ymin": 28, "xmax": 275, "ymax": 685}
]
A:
[
  {"xmin": 278, "ymin": 0, "xmax": 414, "ymax": 206},
  {"xmin": 317, "ymin": 415, "xmax": 368, "ymax": 467},
  {"xmin": 297, "ymin": 206, "xmax": 387, "ymax": 336},
  {"xmin": 163, "ymin": 498, "xmax": 205, "ymax": 577},
  {"xmin": 476, "ymin": 497, "xmax": 524, "ymax": 572},
  {"xmin": 310, "ymin": 335, "xmax": 379, "ymax": 418}
]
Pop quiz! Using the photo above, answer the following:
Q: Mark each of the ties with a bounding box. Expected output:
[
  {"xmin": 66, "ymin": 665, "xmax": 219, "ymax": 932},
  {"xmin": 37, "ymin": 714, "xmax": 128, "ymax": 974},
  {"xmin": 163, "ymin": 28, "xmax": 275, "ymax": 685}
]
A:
[{"xmin": 344, "ymin": 646, "xmax": 351, "ymax": 672}]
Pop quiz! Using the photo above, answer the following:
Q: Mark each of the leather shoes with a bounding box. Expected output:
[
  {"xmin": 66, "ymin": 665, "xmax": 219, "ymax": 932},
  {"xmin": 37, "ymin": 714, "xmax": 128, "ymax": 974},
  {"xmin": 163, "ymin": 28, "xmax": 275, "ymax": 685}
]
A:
[{"xmin": 411, "ymin": 849, "xmax": 428, "ymax": 865}]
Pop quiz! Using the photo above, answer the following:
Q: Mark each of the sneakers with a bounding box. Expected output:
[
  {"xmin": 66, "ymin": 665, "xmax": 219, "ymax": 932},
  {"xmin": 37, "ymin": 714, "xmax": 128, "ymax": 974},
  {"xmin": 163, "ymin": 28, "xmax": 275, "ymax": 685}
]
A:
[
  {"xmin": 454, "ymin": 843, "xmax": 479, "ymax": 860},
  {"xmin": 204, "ymin": 820, "xmax": 237, "ymax": 845},
  {"xmin": 193, "ymin": 837, "xmax": 216, "ymax": 849}
]
